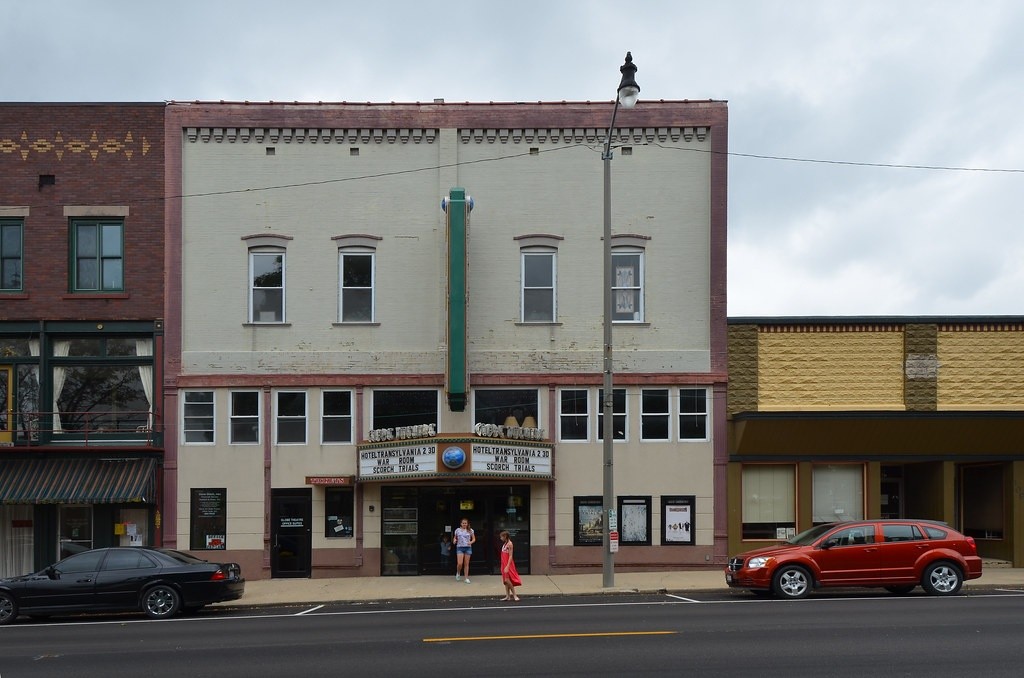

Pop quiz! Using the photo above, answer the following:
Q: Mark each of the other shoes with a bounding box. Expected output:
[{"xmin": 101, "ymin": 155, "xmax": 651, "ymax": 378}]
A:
[
  {"xmin": 500, "ymin": 598, "xmax": 510, "ymax": 601},
  {"xmin": 463, "ymin": 578, "xmax": 470, "ymax": 584},
  {"xmin": 514, "ymin": 596, "xmax": 519, "ymax": 601},
  {"xmin": 456, "ymin": 573, "xmax": 460, "ymax": 581}
]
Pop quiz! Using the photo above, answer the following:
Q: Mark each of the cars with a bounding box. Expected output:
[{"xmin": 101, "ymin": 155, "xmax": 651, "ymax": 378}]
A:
[
  {"xmin": 0, "ymin": 544, "xmax": 245, "ymax": 626},
  {"xmin": 724, "ymin": 519, "xmax": 982, "ymax": 599}
]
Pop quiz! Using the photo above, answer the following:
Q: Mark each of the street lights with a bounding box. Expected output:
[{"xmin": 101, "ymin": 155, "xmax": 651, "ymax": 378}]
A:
[{"xmin": 601, "ymin": 53, "xmax": 641, "ymax": 589}]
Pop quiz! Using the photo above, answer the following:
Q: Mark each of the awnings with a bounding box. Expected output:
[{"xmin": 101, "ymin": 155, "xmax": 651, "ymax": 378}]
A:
[{"xmin": 0, "ymin": 456, "xmax": 158, "ymax": 505}]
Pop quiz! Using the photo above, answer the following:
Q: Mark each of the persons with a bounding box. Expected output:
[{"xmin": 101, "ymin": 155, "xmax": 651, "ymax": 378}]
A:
[
  {"xmin": 499, "ymin": 531, "xmax": 522, "ymax": 602},
  {"xmin": 453, "ymin": 518, "xmax": 476, "ymax": 584},
  {"xmin": 441, "ymin": 534, "xmax": 451, "ymax": 563}
]
